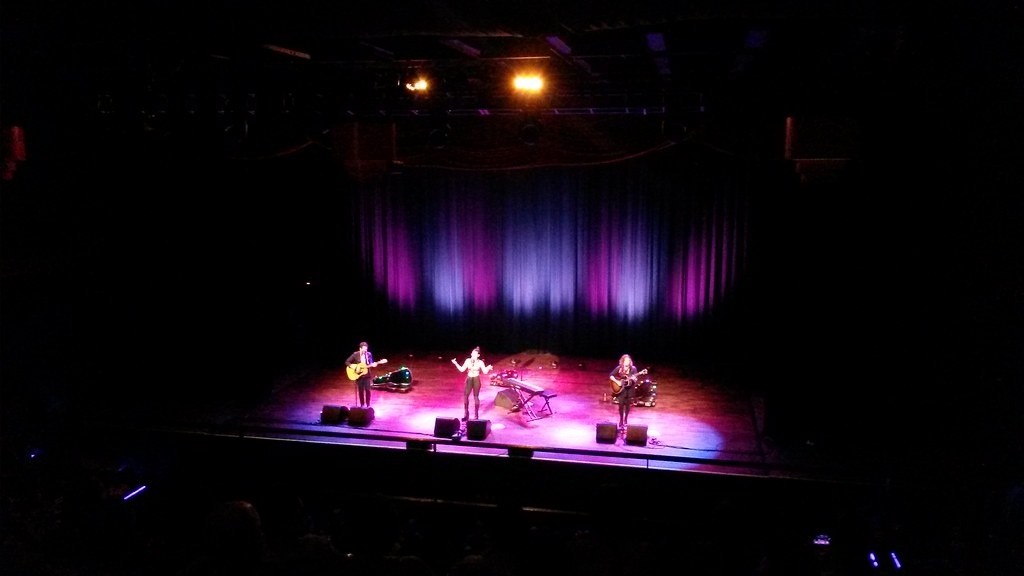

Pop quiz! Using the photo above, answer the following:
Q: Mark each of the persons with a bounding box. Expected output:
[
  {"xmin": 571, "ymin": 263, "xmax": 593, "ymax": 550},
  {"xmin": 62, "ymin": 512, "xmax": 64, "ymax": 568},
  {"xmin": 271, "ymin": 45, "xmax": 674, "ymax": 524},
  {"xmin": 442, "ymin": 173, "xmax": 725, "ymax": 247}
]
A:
[
  {"xmin": 451, "ymin": 347, "xmax": 493, "ymax": 421},
  {"xmin": 344, "ymin": 342, "xmax": 377, "ymax": 408},
  {"xmin": 0, "ymin": 460, "xmax": 1024, "ymax": 576},
  {"xmin": 610, "ymin": 355, "xmax": 637, "ymax": 431}
]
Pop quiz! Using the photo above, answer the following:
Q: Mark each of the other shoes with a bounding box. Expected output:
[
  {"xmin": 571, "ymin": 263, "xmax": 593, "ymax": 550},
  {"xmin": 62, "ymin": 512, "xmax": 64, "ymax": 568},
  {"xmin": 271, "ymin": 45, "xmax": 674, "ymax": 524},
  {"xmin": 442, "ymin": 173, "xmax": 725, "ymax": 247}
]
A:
[
  {"xmin": 618, "ymin": 422, "xmax": 623, "ymax": 429},
  {"xmin": 623, "ymin": 423, "xmax": 627, "ymax": 429},
  {"xmin": 462, "ymin": 415, "xmax": 469, "ymax": 421}
]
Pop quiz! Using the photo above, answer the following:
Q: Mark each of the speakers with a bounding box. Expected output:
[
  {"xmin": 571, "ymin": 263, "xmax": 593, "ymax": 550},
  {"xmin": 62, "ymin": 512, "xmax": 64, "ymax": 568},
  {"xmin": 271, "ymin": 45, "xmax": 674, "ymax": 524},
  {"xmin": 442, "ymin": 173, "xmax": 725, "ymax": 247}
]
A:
[
  {"xmin": 347, "ymin": 406, "xmax": 375, "ymax": 428},
  {"xmin": 321, "ymin": 405, "xmax": 350, "ymax": 425},
  {"xmin": 623, "ymin": 424, "xmax": 648, "ymax": 446},
  {"xmin": 466, "ymin": 419, "xmax": 492, "ymax": 441},
  {"xmin": 596, "ymin": 422, "xmax": 618, "ymax": 444},
  {"xmin": 434, "ymin": 417, "xmax": 461, "ymax": 439}
]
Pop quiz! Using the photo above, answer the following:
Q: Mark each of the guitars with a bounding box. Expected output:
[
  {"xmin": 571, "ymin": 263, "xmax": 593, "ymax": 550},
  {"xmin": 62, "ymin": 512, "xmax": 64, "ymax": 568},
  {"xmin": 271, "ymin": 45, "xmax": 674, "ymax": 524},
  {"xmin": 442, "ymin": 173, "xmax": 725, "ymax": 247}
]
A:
[
  {"xmin": 345, "ymin": 358, "xmax": 389, "ymax": 381},
  {"xmin": 610, "ymin": 368, "xmax": 651, "ymax": 395}
]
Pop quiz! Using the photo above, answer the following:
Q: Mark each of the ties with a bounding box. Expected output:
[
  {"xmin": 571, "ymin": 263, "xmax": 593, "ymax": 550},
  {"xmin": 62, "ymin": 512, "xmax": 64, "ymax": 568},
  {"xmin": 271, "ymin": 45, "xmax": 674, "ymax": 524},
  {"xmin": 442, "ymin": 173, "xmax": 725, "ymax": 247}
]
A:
[{"xmin": 364, "ymin": 353, "xmax": 367, "ymax": 364}]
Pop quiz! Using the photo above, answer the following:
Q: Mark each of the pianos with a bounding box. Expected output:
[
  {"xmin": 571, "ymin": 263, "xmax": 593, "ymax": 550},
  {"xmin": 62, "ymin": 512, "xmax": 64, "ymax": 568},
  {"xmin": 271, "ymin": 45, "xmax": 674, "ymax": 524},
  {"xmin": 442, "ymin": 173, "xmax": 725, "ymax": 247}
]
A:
[{"xmin": 504, "ymin": 377, "xmax": 544, "ymax": 395}]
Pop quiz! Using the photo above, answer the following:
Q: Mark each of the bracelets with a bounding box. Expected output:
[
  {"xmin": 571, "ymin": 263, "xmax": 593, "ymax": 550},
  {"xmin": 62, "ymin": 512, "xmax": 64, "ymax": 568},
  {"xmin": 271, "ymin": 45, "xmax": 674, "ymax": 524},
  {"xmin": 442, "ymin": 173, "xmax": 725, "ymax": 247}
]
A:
[{"xmin": 355, "ymin": 367, "xmax": 357, "ymax": 371}]
